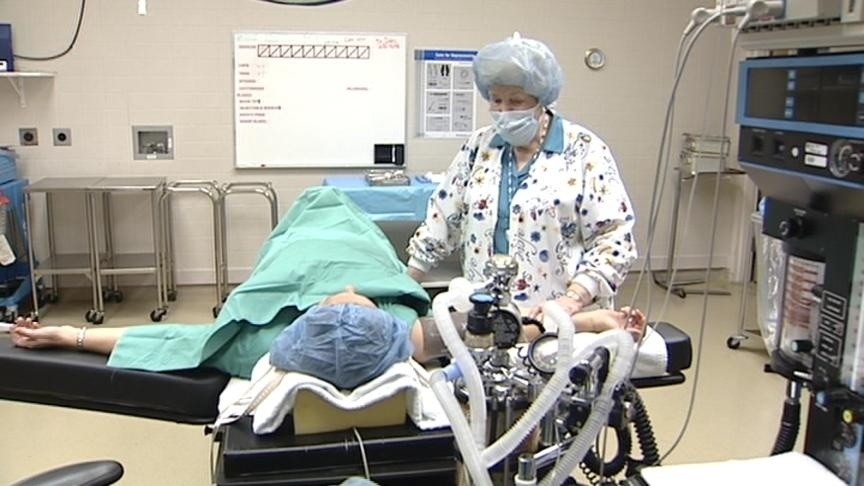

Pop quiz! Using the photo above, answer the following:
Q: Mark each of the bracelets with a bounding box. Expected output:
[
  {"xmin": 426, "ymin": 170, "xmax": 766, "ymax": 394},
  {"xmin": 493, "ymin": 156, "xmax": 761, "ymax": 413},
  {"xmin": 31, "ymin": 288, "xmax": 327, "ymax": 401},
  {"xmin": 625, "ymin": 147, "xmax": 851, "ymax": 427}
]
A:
[
  {"xmin": 77, "ymin": 326, "xmax": 87, "ymax": 350},
  {"xmin": 557, "ymin": 287, "xmax": 585, "ymax": 308}
]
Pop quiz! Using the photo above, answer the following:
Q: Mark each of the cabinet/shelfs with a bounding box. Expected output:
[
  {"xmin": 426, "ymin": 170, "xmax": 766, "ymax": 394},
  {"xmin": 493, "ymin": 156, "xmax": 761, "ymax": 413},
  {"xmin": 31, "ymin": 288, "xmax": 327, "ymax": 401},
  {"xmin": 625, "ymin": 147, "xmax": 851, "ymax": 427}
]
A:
[{"xmin": 15, "ymin": 174, "xmax": 170, "ymax": 324}]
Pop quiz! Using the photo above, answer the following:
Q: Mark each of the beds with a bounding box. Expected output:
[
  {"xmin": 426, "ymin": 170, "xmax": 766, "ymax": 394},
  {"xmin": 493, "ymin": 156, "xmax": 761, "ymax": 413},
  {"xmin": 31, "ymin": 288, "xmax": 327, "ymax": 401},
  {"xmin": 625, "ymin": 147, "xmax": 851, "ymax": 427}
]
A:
[{"xmin": 0, "ymin": 185, "xmax": 696, "ymax": 486}]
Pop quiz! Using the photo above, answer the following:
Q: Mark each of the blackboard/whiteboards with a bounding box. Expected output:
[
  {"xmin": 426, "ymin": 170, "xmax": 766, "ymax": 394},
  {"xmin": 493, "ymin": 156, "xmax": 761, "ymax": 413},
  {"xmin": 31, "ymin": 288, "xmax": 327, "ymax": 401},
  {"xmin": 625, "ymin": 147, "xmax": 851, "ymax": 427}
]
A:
[{"xmin": 234, "ymin": 31, "xmax": 410, "ymax": 171}]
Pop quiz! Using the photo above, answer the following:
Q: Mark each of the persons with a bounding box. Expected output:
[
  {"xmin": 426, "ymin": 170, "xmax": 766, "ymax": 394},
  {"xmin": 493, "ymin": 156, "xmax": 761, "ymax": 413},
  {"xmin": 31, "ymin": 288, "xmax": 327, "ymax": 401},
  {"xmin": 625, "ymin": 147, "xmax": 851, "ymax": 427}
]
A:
[
  {"xmin": 7, "ymin": 280, "xmax": 650, "ymax": 394},
  {"xmin": 402, "ymin": 30, "xmax": 638, "ymax": 341}
]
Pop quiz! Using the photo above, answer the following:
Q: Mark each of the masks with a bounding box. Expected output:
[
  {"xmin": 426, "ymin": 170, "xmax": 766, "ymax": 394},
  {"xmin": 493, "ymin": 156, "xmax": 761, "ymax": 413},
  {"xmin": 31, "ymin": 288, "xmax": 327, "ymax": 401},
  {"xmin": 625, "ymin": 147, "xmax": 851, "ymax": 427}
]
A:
[{"xmin": 488, "ymin": 104, "xmax": 545, "ymax": 146}]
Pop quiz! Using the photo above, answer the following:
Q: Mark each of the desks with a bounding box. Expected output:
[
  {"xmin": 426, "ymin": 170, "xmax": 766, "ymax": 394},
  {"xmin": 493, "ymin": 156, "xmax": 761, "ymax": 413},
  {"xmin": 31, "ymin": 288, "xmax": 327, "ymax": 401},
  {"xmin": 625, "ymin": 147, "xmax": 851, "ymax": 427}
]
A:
[{"xmin": 324, "ymin": 174, "xmax": 460, "ymax": 221}]
